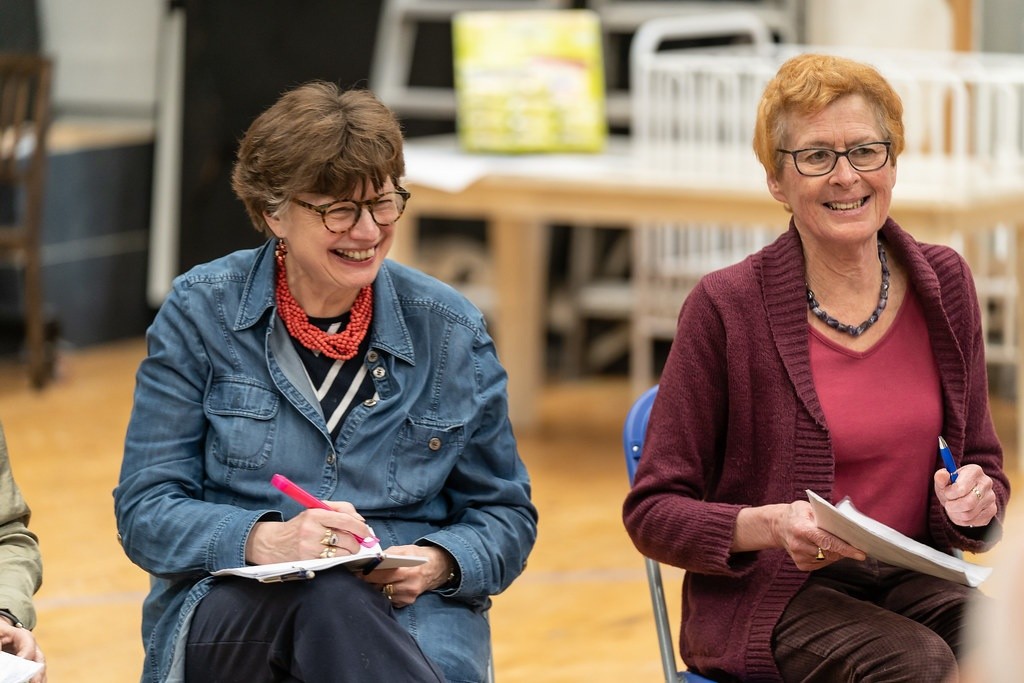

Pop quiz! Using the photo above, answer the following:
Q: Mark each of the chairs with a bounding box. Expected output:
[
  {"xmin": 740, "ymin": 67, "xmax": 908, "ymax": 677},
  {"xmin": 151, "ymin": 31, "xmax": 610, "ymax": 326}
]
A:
[
  {"xmin": 626, "ymin": 386, "xmax": 735, "ymax": 683},
  {"xmin": 0, "ymin": 51, "xmax": 51, "ymax": 388}
]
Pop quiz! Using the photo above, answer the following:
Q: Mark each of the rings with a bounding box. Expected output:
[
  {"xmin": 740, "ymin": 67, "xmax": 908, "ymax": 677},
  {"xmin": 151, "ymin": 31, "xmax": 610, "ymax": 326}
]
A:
[
  {"xmin": 319, "ymin": 548, "xmax": 336, "ymax": 558},
  {"xmin": 275, "ymin": 239, "xmax": 374, "ymax": 361},
  {"xmin": 971, "ymin": 487, "xmax": 983, "ymax": 501},
  {"xmin": 388, "ymin": 596, "xmax": 393, "ymax": 600},
  {"xmin": 816, "ymin": 547, "xmax": 825, "ymax": 561},
  {"xmin": 320, "ymin": 528, "xmax": 338, "ymax": 545},
  {"xmin": 387, "ymin": 585, "xmax": 393, "ymax": 595}
]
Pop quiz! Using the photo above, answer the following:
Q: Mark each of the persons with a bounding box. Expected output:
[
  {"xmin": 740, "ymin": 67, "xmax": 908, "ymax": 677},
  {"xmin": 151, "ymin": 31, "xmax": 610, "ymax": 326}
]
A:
[
  {"xmin": 112, "ymin": 78, "xmax": 541, "ymax": 682},
  {"xmin": 623, "ymin": 56, "xmax": 1013, "ymax": 682},
  {"xmin": 0, "ymin": 420, "xmax": 48, "ymax": 683}
]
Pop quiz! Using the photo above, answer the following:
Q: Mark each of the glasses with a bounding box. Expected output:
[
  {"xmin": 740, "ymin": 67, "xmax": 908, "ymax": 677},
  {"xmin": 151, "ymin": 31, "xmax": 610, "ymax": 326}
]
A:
[
  {"xmin": 278, "ymin": 184, "xmax": 410, "ymax": 234},
  {"xmin": 777, "ymin": 140, "xmax": 892, "ymax": 178}
]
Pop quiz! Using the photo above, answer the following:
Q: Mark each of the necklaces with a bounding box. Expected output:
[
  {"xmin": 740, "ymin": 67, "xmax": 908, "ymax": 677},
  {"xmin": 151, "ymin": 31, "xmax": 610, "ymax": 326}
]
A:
[{"xmin": 805, "ymin": 239, "xmax": 891, "ymax": 335}]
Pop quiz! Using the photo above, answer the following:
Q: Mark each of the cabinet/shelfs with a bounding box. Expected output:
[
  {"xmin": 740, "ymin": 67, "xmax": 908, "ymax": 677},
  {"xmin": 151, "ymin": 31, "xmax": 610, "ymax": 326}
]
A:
[{"xmin": 629, "ymin": 46, "xmax": 1023, "ymax": 378}]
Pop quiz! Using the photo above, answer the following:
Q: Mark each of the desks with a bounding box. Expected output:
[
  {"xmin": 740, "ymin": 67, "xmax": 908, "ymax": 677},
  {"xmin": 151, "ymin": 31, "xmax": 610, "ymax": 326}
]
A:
[{"xmin": 391, "ymin": 129, "xmax": 1023, "ymax": 430}]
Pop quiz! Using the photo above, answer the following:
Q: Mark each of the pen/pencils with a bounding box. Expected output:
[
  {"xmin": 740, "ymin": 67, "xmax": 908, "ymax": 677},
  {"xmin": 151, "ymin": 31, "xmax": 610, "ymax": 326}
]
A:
[
  {"xmin": 363, "ymin": 556, "xmax": 384, "ymax": 576},
  {"xmin": 261, "ymin": 570, "xmax": 315, "ymax": 584},
  {"xmin": 939, "ymin": 436, "xmax": 958, "ymax": 483},
  {"xmin": 271, "ymin": 474, "xmax": 380, "ymax": 548}
]
